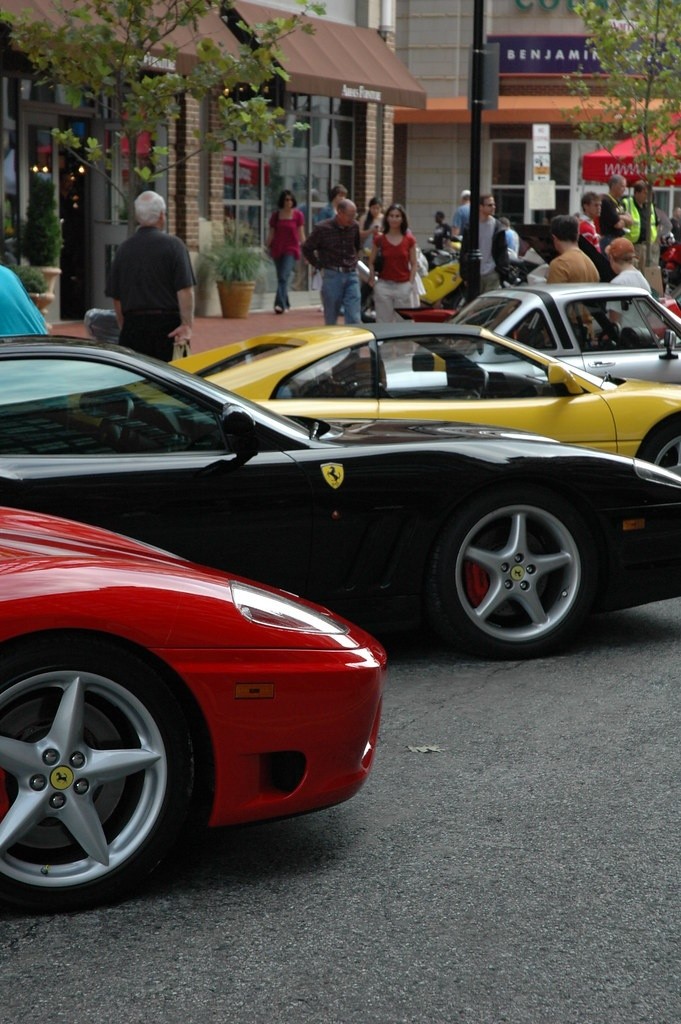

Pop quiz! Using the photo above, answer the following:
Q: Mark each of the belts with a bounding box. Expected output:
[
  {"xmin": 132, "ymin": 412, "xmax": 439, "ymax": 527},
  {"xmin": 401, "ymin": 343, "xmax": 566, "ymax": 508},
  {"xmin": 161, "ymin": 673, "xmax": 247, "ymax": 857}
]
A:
[
  {"xmin": 126, "ymin": 310, "xmax": 162, "ymax": 314},
  {"xmin": 326, "ymin": 266, "xmax": 356, "ymax": 273}
]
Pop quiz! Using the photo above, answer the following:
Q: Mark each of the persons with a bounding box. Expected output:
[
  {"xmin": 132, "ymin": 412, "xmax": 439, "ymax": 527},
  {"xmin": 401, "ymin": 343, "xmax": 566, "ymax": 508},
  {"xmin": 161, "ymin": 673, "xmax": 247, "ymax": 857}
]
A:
[
  {"xmin": 369, "ymin": 204, "xmax": 417, "ymax": 323},
  {"xmin": 459, "ymin": 194, "xmax": 511, "ymax": 295},
  {"xmin": 0, "ymin": 264, "xmax": 51, "ymax": 334},
  {"xmin": 266, "ymin": 190, "xmax": 306, "ymax": 313},
  {"xmin": 318, "ymin": 184, "xmax": 422, "ymax": 315},
  {"xmin": 110, "ymin": 191, "xmax": 197, "ymax": 363},
  {"xmin": 300, "ymin": 199, "xmax": 362, "ymax": 326},
  {"xmin": 498, "ymin": 174, "xmax": 681, "ymax": 297},
  {"xmin": 434, "ymin": 210, "xmax": 452, "ymax": 234},
  {"xmin": 453, "ymin": 190, "xmax": 470, "ymax": 235}
]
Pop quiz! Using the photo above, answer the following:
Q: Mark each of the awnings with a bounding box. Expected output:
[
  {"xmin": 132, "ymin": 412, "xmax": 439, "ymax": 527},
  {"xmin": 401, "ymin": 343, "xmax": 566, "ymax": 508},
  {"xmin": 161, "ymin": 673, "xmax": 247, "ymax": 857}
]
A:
[
  {"xmin": 229, "ymin": 0, "xmax": 427, "ymax": 109},
  {"xmin": 0, "ymin": 0, "xmax": 263, "ymax": 85}
]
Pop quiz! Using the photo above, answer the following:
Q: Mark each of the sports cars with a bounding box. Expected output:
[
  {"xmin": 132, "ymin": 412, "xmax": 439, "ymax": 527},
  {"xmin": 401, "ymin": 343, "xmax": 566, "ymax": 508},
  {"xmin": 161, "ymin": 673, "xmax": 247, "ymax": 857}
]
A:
[
  {"xmin": 0, "ymin": 507, "xmax": 388, "ymax": 918},
  {"xmin": 0, "ymin": 335, "xmax": 681, "ymax": 660},
  {"xmin": 67, "ymin": 324, "xmax": 680, "ymax": 480}
]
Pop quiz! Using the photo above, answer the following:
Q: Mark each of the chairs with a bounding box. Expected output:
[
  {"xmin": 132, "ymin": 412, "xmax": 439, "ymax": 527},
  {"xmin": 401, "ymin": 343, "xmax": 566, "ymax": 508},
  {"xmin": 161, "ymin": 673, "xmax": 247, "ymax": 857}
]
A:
[
  {"xmin": 331, "ymin": 352, "xmax": 361, "ymax": 396},
  {"xmin": 353, "ymin": 357, "xmax": 387, "ymax": 398}
]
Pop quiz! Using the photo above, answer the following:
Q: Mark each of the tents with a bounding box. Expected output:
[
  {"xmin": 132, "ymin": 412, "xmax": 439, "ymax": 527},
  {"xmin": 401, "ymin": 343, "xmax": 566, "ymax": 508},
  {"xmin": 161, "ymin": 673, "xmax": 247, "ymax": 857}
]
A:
[{"xmin": 581, "ymin": 112, "xmax": 681, "ymax": 219}]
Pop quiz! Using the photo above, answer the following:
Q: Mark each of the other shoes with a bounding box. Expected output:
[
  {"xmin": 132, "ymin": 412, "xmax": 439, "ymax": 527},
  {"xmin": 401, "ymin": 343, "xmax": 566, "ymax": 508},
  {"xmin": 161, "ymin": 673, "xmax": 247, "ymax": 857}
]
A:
[{"xmin": 273, "ymin": 304, "xmax": 289, "ymax": 314}]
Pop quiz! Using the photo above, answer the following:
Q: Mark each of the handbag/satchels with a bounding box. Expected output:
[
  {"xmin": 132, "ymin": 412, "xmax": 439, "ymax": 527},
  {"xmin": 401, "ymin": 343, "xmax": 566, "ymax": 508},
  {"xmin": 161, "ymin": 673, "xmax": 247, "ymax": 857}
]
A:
[{"xmin": 373, "ymin": 234, "xmax": 384, "ymax": 273}]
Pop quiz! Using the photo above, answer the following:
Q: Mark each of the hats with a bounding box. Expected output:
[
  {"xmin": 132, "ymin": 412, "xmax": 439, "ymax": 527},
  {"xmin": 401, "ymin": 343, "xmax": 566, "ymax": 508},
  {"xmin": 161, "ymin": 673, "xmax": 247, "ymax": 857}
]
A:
[{"xmin": 605, "ymin": 238, "xmax": 638, "ymax": 260}]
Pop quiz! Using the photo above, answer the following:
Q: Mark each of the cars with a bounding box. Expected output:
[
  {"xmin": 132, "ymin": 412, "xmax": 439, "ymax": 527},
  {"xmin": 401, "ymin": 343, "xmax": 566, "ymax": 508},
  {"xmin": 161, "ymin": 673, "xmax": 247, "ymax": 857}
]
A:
[{"xmin": 384, "ymin": 283, "xmax": 680, "ymax": 389}]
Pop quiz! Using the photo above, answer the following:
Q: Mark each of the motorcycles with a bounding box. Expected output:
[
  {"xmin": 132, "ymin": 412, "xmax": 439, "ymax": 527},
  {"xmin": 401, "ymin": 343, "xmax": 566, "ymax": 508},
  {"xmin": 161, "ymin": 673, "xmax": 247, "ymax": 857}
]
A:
[{"xmin": 357, "ymin": 232, "xmax": 463, "ymax": 325}]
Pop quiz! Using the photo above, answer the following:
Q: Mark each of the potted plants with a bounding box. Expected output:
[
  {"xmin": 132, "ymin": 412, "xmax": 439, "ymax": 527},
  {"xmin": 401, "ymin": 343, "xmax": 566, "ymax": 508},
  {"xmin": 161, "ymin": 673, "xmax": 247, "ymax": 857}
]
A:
[
  {"xmin": 12, "ymin": 172, "xmax": 65, "ymax": 292},
  {"xmin": 6, "ymin": 263, "xmax": 56, "ymax": 315},
  {"xmin": 199, "ymin": 217, "xmax": 274, "ymax": 319}
]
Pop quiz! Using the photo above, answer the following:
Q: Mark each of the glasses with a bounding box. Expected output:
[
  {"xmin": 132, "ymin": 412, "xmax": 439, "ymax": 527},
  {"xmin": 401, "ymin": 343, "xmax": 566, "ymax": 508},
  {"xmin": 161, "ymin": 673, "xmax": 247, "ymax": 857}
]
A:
[{"xmin": 482, "ymin": 203, "xmax": 495, "ymax": 208}]
